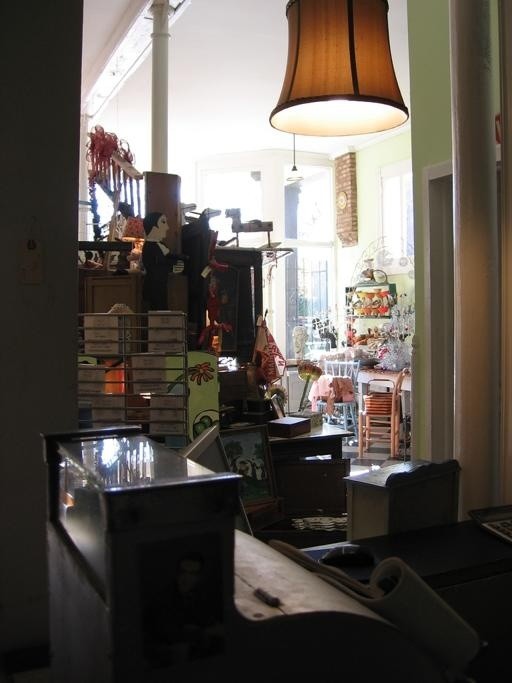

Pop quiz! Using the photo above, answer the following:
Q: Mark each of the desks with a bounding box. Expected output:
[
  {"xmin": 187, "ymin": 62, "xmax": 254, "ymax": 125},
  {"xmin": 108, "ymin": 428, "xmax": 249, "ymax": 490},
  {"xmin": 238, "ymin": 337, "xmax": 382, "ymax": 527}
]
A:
[{"xmin": 357, "ymin": 368, "xmax": 411, "ymax": 411}]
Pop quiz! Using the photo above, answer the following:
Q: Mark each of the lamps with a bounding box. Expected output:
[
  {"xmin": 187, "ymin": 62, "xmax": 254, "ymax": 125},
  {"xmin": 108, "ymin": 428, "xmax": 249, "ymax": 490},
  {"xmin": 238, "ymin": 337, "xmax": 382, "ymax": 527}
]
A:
[
  {"xmin": 287, "ymin": 133, "xmax": 302, "ymax": 181},
  {"xmin": 268, "ymin": 0, "xmax": 408, "ymax": 135}
]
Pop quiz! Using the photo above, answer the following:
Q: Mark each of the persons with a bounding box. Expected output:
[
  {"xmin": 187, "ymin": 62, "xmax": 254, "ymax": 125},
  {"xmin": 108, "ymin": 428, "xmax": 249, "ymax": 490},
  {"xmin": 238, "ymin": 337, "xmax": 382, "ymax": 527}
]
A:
[
  {"xmin": 142, "ymin": 551, "xmax": 225, "ymax": 669},
  {"xmin": 141, "ymin": 212, "xmax": 176, "ymax": 353}
]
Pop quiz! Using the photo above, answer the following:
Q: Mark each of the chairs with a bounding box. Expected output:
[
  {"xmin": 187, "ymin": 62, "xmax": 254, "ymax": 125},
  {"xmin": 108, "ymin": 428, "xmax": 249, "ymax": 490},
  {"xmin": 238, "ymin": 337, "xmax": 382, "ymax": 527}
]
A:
[
  {"xmin": 317, "ymin": 360, "xmax": 360, "ymax": 445},
  {"xmin": 358, "ymin": 368, "xmax": 406, "ymax": 458}
]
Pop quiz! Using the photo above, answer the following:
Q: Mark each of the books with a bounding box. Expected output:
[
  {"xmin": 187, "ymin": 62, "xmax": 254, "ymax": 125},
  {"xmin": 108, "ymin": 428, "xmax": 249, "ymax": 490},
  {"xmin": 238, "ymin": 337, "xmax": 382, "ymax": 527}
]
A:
[{"xmin": 269, "ymin": 538, "xmax": 480, "ymax": 668}]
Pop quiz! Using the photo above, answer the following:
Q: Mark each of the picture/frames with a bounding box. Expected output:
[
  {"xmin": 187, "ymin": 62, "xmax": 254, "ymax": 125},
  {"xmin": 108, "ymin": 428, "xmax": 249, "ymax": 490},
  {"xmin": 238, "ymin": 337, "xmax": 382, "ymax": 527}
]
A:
[
  {"xmin": 78, "ymin": 268, "xmax": 145, "ymax": 356},
  {"xmin": 220, "ymin": 423, "xmax": 284, "ymax": 530}
]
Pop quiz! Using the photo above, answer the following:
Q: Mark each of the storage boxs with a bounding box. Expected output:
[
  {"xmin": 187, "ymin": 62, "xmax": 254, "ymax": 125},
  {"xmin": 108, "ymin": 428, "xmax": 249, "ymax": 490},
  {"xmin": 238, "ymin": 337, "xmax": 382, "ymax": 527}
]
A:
[
  {"xmin": 267, "ymin": 412, "xmax": 322, "ymax": 437},
  {"xmin": 79, "ymin": 310, "xmax": 185, "ymax": 434}
]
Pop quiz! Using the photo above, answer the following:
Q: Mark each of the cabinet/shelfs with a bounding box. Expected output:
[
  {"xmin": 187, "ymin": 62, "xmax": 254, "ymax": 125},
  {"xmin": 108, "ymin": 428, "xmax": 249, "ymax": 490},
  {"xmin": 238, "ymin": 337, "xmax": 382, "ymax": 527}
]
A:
[
  {"xmin": 346, "ymin": 282, "xmax": 397, "ymax": 347},
  {"xmin": 39, "ymin": 425, "xmax": 243, "ymax": 683},
  {"xmin": 268, "ymin": 422, "xmax": 355, "ymax": 517},
  {"xmin": 342, "ymin": 459, "xmax": 462, "ymax": 541}
]
